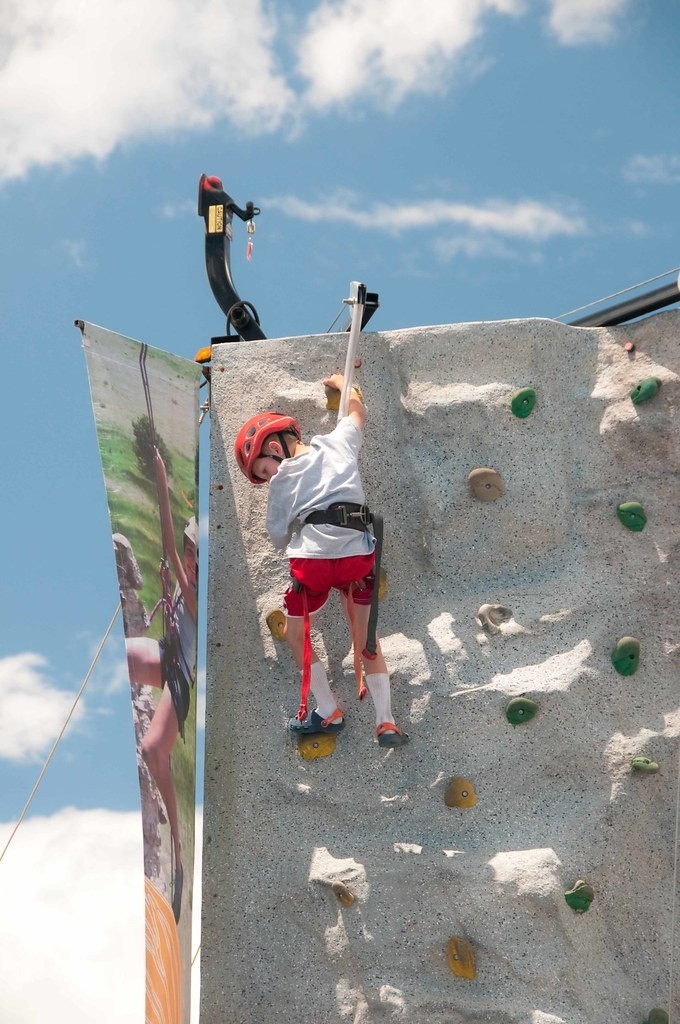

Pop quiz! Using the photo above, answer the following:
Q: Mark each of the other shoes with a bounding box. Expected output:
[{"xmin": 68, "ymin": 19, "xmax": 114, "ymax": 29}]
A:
[{"xmin": 171, "ymin": 863, "xmax": 184, "ymax": 924}]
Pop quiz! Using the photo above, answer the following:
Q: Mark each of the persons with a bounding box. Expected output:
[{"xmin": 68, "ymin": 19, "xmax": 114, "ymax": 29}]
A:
[
  {"xmin": 125, "ymin": 448, "xmax": 199, "ymax": 922},
  {"xmin": 235, "ymin": 374, "xmax": 410, "ymax": 747}
]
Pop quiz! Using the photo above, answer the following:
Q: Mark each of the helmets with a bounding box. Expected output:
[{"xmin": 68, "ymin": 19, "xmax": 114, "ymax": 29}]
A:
[
  {"xmin": 235, "ymin": 412, "xmax": 302, "ymax": 485},
  {"xmin": 183, "ymin": 515, "xmax": 197, "ymax": 546}
]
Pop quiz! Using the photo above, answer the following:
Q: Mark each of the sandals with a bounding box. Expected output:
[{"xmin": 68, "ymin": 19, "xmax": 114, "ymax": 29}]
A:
[
  {"xmin": 376, "ymin": 723, "xmax": 412, "ymax": 748},
  {"xmin": 289, "ymin": 707, "xmax": 345, "ymax": 733}
]
